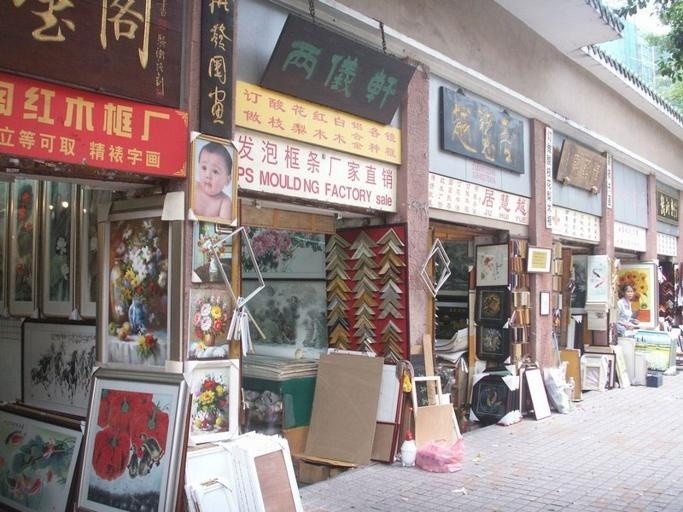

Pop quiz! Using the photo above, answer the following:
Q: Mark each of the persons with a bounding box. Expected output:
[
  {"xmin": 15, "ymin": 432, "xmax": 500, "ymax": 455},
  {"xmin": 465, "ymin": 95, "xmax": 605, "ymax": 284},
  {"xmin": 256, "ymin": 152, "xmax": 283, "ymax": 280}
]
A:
[
  {"xmin": 617, "ymin": 283, "xmax": 643, "ymax": 340},
  {"xmin": 193, "ymin": 134, "xmax": 235, "ymax": 220}
]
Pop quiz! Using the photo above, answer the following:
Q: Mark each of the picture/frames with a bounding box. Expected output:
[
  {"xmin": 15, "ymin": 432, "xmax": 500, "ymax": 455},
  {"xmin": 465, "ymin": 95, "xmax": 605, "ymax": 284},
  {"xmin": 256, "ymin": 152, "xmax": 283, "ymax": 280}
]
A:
[
  {"xmin": 554, "ymin": 247, "xmax": 678, "ymax": 403},
  {"xmin": 306, "ymin": 344, "xmax": 462, "ymax": 466},
  {"xmin": 0, "ymin": 174, "xmax": 327, "ymax": 512},
  {"xmin": 432, "ymin": 234, "xmax": 551, "ymax": 427}
]
